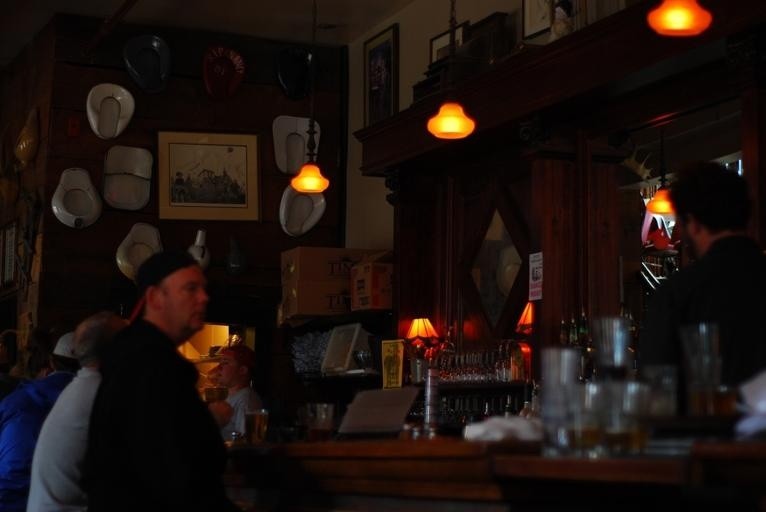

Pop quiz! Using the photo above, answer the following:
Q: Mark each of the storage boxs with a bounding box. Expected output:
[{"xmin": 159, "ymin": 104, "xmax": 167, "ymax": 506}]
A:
[{"xmin": 280, "ymin": 246, "xmax": 390, "ymax": 320}]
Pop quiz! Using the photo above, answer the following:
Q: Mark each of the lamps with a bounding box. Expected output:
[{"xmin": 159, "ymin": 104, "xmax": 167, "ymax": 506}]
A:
[
  {"xmin": 426, "ymin": 1, "xmax": 476, "ymax": 141},
  {"xmin": 646, "ymin": 1, "xmax": 713, "ymax": 38},
  {"xmin": 405, "ymin": 316, "xmax": 438, "ymax": 345},
  {"xmin": 515, "ymin": 301, "xmax": 536, "ymax": 335},
  {"xmin": 646, "ymin": 126, "xmax": 675, "ymax": 214},
  {"xmin": 288, "ymin": 0, "xmax": 331, "ymax": 195}
]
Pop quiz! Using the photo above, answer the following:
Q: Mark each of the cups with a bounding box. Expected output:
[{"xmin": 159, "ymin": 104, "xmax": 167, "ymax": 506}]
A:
[
  {"xmin": 316, "ymin": 403, "xmax": 333, "ymax": 428},
  {"xmin": 533, "ymin": 314, "xmax": 735, "ymax": 460},
  {"xmin": 243, "ymin": 407, "xmax": 268, "ymax": 447}
]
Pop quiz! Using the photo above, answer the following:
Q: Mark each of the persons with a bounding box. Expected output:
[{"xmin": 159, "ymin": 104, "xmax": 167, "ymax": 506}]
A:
[
  {"xmin": 1, "ymin": 246, "xmax": 226, "ymax": 512},
  {"xmin": 636, "ymin": 163, "xmax": 766, "ymax": 407},
  {"xmin": 202, "ymin": 345, "xmax": 262, "ymax": 441}
]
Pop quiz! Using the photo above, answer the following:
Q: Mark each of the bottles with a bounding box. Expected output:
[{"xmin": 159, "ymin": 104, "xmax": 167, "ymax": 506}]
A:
[{"xmin": 406, "ymin": 343, "xmax": 532, "ymax": 428}]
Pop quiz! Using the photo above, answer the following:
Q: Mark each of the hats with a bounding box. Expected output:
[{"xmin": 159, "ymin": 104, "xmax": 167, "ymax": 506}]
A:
[
  {"xmin": 122, "ymin": 247, "xmax": 203, "ymax": 326},
  {"xmin": 52, "ymin": 332, "xmax": 97, "ymax": 361}
]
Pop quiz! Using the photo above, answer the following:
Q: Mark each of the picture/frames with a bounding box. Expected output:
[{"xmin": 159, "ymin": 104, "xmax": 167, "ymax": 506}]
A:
[
  {"xmin": 157, "ymin": 130, "xmax": 261, "ymax": 223},
  {"xmin": 430, "ymin": 20, "xmax": 470, "ymax": 66},
  {"xmin": 362, "ymin": 23, "xmax": 400, "ymax": 130},
  {"xmin": 521, "ymin": 0, "xmax": 574, "ymax": 40}
]
